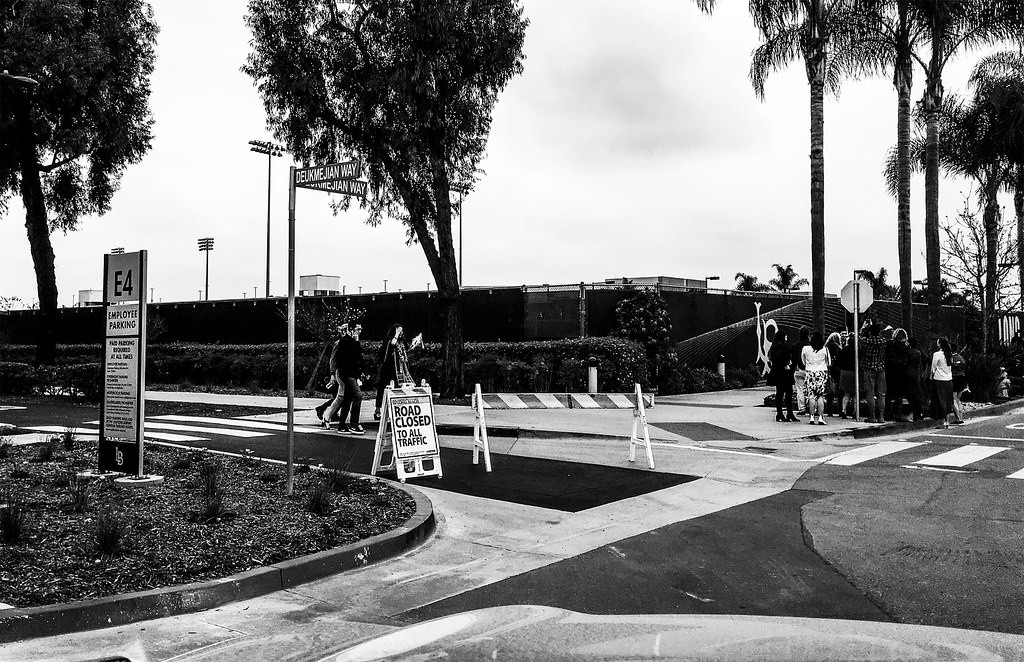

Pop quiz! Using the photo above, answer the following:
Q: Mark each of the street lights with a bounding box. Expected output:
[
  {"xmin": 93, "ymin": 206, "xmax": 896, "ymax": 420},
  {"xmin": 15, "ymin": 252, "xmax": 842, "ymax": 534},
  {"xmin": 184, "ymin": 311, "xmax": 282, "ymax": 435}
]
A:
[
  {"xmin": 249, "ymin": 139, "xmax": 287, "ymax": 299},
  {"xmin": 704, "ymin": 275, "xmax": 720, "ymax": 293},
  {"xmin": 457, "ymin": 180, "xmax": 469, "ymax": 286},
  {"xmin": 197, "ymin": 236, "xmax": 214, "ymax": 300}
]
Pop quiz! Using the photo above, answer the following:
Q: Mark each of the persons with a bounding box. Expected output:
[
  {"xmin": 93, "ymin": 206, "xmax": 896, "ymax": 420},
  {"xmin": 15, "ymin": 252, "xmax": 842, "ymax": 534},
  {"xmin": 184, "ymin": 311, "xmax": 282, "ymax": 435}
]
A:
[
  {"xmin": 769, "ymin": 319, "xmax": 970, "ymax": 429},
  {"xmin": 316, "ymin": 320, "xmax": 416, "ymax": 434}
]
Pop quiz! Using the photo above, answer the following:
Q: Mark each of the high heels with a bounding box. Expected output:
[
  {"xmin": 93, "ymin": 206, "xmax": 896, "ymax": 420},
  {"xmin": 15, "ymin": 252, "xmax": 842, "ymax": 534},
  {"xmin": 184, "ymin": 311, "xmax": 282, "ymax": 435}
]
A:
[
  {"xmin": 786, "ymin": 414, "xmax": 800, "ymax": 422},
  {"xmin": 776, "ymin": 415, "xmax": 787, "ymax": 422}
]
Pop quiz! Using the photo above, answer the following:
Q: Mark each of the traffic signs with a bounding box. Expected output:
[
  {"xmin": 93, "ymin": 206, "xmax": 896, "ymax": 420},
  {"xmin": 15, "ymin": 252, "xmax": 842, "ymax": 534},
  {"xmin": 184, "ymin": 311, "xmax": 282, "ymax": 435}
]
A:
[
  {"xmin": 297, "ymin": 178, "xmax": 368, "ymax": 198},
  {"xmin": 293, "ymin": 160, "xmax": 361, "ymax": 186}
]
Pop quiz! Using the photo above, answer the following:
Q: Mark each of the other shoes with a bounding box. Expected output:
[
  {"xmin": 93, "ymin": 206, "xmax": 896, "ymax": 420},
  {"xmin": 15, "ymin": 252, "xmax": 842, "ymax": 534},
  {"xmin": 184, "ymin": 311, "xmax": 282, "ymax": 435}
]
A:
[
  {"xmin": 374, "ymin": 414, "xmax": 381, "ymax": 420},
  {"xmin": 797, "ymin": 410, "xmax": 806, "ymax": 415},
  {"xmin": 331, "ymin": 413, "xmax": 340, "ymax": 422},
  {"xmin": 322, "ymin": 418, "xmax": 330, "ymax": 430},
  {"xmin": 852, "ymin": 413, "xmax": 856, "ymax": 419},
  {"xmin": 877, "ymin": 418, "xmax": 884, "ymax": 423},
  {"xmin": 935, "ymin": 422, "xmax": 950, "ymax": 429},
  {"xmin": 315, "ymin": 406, "xmax": 323, "ymax": 420},
  {"xmin": 349, "ymin": 425, "xmax": 366, "ymax": 434},
  {"xmin": 864, "ymin": 418, "xmax": 876, "ymax": 423},
  {"xmin": 337, "ymin": 426, "xmax": 351, "ymax": 434},
  {"xmin": 818, "ymin": 420, "xmax": 827, "ymax": 425},
  {"xmin": 949, "ymin": 421, "xmax": 964, "ymax": 425},
  {"xmin": 809, "ymin": 420, "xmax": 814, "ymax": 424},
  {"xmin": 842, "ymin": 412, "xmax": 848, "ymax": 419}
]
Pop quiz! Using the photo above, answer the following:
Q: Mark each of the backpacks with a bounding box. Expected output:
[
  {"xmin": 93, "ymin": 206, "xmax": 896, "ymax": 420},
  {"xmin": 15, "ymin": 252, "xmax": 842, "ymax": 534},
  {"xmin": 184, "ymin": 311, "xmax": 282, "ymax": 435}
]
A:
[{"xmin": 951, "ymin": 354, "xmax": 968, "ymax": 392}]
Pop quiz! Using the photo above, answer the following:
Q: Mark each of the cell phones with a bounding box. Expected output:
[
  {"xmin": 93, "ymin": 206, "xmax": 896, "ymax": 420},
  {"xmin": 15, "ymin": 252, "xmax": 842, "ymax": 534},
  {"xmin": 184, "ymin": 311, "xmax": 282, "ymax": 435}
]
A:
[{"xmin": 866, "ymin": 319, "xmax": 870, "ymax": 327}]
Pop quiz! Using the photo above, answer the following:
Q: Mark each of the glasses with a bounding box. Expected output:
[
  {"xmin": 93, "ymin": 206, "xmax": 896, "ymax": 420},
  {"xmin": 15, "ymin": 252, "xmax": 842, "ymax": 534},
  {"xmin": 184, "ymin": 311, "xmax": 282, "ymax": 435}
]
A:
[{"xmin": 355, "ymin": 328, "xmax": 363, "ymax": 331}]
